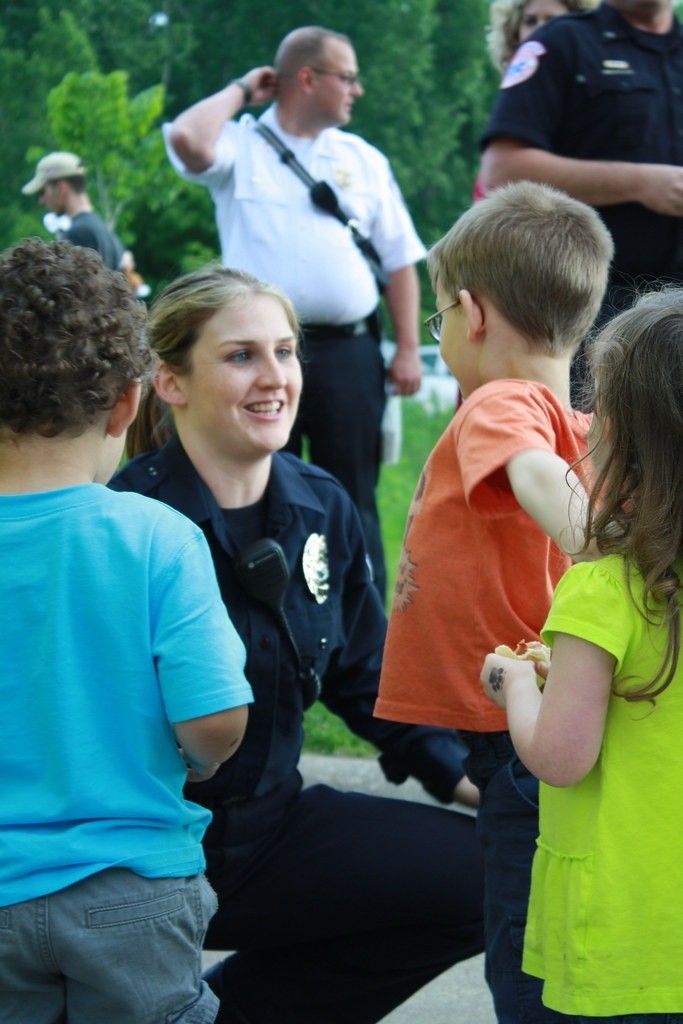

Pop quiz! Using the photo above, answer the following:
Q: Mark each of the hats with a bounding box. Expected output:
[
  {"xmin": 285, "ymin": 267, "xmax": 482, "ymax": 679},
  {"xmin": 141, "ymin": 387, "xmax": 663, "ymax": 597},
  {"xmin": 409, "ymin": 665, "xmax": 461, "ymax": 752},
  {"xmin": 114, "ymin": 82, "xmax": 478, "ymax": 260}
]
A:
[{"xmin": 21, "ymin": 152, "xmax": 87, "ymax": 195}]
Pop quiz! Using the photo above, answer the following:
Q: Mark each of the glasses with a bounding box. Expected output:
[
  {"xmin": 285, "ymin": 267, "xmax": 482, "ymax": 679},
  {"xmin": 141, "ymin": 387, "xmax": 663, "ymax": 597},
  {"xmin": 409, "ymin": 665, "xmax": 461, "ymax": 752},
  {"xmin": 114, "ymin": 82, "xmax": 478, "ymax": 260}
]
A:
[
  {"xmin": 310, "ymin": 66, "xmax": 360, "ymax": 82},
  {"xmin": 423, "ymin": 295, "xmax": 488, "ymax": 345}
]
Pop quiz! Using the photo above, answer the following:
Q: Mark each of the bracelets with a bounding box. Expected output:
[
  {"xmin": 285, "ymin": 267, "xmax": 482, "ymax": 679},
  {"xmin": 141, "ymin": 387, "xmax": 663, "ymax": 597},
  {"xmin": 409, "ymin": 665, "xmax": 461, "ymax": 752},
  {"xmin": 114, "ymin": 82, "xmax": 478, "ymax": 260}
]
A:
[{"xmin": 229, "ymin": 77, "xmax": 253, "ymax": 106}]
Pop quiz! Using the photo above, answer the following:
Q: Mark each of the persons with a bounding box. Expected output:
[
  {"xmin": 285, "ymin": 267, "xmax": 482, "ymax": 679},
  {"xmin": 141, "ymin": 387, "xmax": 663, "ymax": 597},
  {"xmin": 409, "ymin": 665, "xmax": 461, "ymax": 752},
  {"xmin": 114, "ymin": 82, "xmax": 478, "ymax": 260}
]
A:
[
  {"xmin": 20, "ymin": 152, "xmax": 123, "ymax": 274},
  {"xmin": 484, "ymin": 0, "xmax": 601, "ymax": 76},
  {"xmin": 479, "ymin": 280, "xmax": 683, "ymax": 1024},
  {"xmin": 106, "ymin": 265, "xmax": 482, "ymax": 1024},
  {"xmin": 155, "ymin": 27, "xmax": 432, "ymax": 611},
  {"xmin": 368, "ymin": 181, "xmax": 624, "ymax": 1024},
  {"xmin": 0, "ymin": 235, "xmax": 260, "ymax": 1024},
  {"xmin": 470, "ymin": 0, "xmax": 683, "ymax": 412},
  {"xmin": 116, "ymin": 249, "xmax": 149, "ymax": 300}
]
